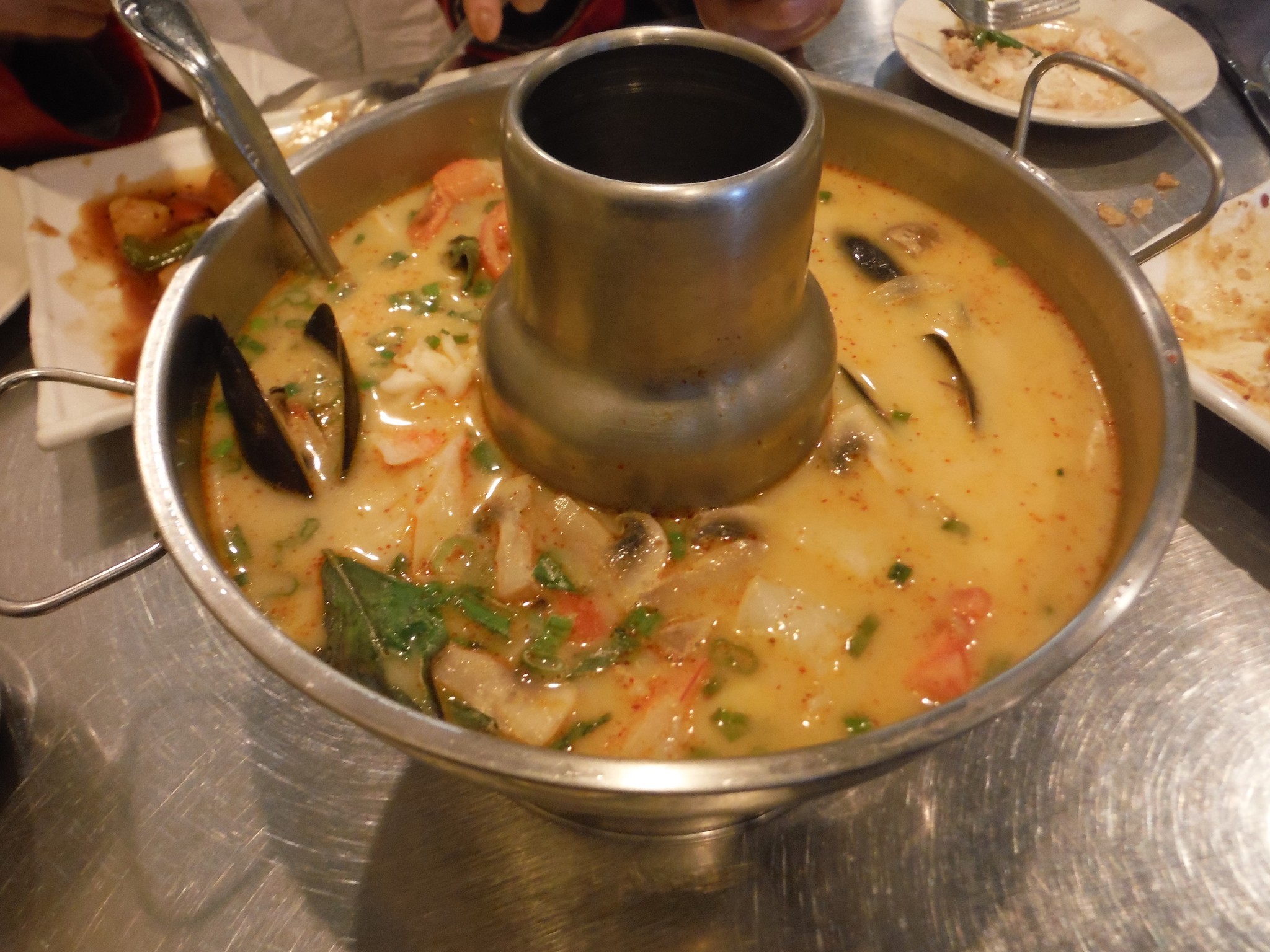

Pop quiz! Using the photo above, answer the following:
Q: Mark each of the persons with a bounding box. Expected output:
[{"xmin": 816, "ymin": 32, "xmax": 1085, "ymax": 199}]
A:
[{"xmin": 2, "ymin": 0, "xmax": 848, "ymax": 169}]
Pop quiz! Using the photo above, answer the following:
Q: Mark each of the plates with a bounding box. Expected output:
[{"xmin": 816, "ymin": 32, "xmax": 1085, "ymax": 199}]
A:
[
  {"xmin": 26, "ymin": 65, "xmax": 484, "ymax": 448},
  {"xmin": 1136, "ymin": 173, "xmax": 1270, "ymax": 468},
  {"xmin": 890, "ymin": 0, "xmax": 1218, "ymax": 133}
]
